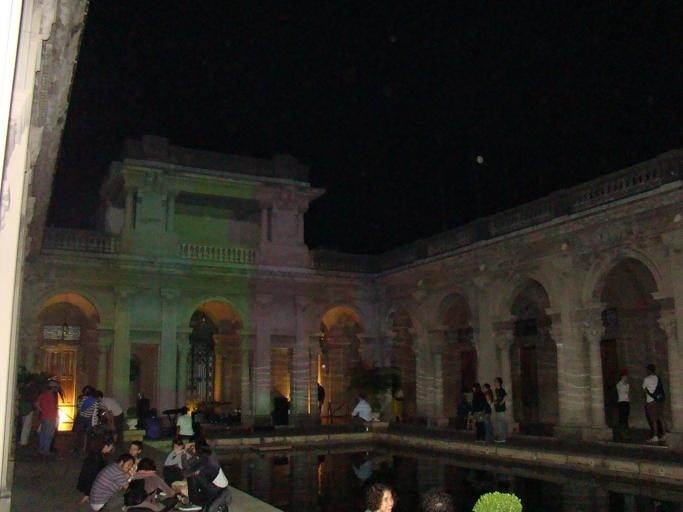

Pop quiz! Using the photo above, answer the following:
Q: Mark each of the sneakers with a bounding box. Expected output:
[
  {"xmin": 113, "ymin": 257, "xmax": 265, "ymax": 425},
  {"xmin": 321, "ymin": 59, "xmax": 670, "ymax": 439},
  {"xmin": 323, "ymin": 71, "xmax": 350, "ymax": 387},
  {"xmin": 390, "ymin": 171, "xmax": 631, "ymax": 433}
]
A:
[
  {"xmin": 658, "ymin": 435, "xmax": 665, "ymax": 441},
  {"xmin": 180, "ymin": 503, "xmax": 201, "ymax": 511},
  {"xmin": 648, "ymin": 436, "xmax": 659, "ymax": 442}
]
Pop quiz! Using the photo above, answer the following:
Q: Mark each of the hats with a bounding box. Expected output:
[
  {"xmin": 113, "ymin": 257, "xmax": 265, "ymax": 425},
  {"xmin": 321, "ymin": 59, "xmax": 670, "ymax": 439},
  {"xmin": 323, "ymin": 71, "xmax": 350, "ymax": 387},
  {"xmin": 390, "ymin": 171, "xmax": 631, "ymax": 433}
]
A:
[
  {"xmin": 49, "ymin": 381, "xmax": 57, "ymax": 387},
  {"xmin": 645, "ymin": 364, "xmax": 656, "ymax": 370}
]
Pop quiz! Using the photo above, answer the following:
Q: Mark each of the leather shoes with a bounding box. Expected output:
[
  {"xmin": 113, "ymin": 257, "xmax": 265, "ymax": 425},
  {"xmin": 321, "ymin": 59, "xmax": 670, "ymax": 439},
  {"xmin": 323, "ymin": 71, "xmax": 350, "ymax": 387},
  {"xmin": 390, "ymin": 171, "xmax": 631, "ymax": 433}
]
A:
[{"xmin": 77, "ymin": 496, "xmax": 89, "ymax": 504}]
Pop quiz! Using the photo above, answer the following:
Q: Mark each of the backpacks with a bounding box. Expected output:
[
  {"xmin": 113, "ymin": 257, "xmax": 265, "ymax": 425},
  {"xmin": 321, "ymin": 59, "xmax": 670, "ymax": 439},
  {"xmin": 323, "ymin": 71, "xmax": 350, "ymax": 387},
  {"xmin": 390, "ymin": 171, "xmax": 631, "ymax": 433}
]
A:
[
  {"xmin": 124, "ymin": 479, "xmax": 155, "ymax": 506},
  {"xmin": 649, "ymin": 377, "xmax": 666, "ymax": 401}
]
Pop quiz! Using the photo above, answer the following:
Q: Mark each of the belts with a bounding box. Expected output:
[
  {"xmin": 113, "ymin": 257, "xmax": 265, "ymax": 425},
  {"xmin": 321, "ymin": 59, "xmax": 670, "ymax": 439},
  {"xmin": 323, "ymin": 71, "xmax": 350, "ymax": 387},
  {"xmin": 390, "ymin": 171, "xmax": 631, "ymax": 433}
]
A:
[{"xmin": 98, "ymin": 503, "xmax": 106, "ymax": 511}]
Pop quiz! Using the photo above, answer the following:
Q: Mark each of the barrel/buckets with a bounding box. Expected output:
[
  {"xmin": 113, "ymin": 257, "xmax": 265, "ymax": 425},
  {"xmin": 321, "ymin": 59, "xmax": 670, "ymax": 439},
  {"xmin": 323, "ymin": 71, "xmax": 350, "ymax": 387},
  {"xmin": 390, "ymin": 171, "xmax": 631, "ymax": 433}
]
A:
[{"xmin": 144, "ymin": 416, "xmax": 162, "ymax": 438}]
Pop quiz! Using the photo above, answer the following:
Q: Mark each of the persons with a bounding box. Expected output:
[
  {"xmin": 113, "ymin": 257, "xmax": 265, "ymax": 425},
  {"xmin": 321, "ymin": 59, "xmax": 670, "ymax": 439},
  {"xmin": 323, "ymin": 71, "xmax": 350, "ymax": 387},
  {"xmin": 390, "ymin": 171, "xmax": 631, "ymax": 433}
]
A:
[
  {"xmin": 348, "ymin": 392, "xmax": 372, "ymax": 433},
  {"xmin": 316, "ymin": 383, "xmax": 324, "ymax": 416},
  {"xmin": 612, "ymin": 372, "xmax": 633, "ymax": 442},
  {"xmin": 468, "ymin": 376, "xmax": 510, "ymax": 444},
  {"xmin": 16, "ymin": 372, "xmax": 63, "ymax": 456},
  {"xmin": 362, "ymin": 482, "xmax": 395, "ymax": 512},
  {"xmin": 68, "ymin": 385, "xmax": 232, "ymax": 512},
  {"xmin": 351, "ymin": 455, "xmax": 375, "ymax": 487},
  {"xmin": 270, "ymin": 394, "xmax": 289, "ymax": 425},
  {"xmin": 640, "ymin": 364, "xmax": 667, "ymax": 443}
]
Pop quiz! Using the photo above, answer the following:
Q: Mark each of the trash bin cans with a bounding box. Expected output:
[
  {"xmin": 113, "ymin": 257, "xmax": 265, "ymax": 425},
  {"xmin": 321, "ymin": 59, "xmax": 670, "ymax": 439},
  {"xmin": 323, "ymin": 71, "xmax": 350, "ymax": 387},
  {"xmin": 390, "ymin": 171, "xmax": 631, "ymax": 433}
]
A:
[{"xmin": 137, "ymin": 400, "xmax": 151, "ymax": 416}]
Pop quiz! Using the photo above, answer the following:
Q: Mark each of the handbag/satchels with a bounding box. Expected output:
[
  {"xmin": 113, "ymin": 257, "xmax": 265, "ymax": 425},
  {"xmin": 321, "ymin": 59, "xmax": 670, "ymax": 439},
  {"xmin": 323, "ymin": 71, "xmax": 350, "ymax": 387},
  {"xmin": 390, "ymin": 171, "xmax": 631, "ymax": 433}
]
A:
[{"xmin": 189, "ymin": 471, "xmax": 209, "ymax": 504}]
